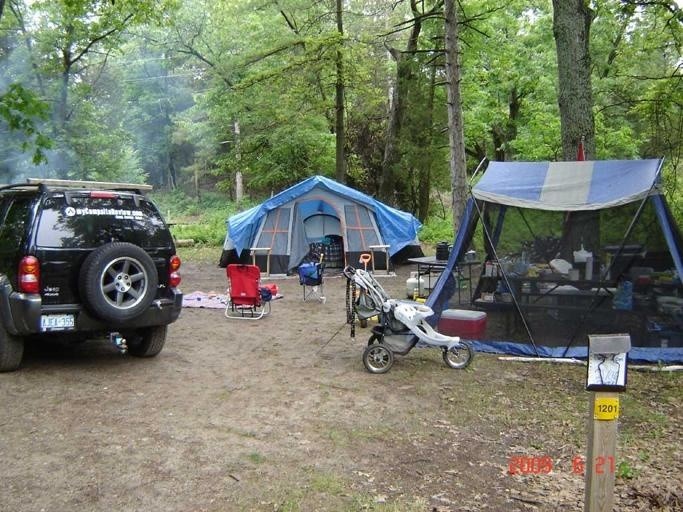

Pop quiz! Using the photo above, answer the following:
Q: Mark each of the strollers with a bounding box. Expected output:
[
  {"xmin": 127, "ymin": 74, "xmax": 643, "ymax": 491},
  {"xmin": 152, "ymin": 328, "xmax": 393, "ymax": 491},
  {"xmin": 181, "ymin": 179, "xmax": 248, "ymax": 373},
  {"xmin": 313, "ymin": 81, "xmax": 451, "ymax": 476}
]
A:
[{"xmin": 341, "ymin": 265, "xmax": 475, "ymax": 375}]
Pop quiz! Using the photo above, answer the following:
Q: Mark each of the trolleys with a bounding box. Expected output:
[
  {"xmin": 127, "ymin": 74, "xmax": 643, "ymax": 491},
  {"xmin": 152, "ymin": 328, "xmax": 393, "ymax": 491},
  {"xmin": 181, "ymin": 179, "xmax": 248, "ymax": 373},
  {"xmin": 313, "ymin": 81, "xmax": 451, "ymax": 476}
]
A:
[{"xmin": 346, "ymin": 253, "xmax": 382, "ymax": 328}]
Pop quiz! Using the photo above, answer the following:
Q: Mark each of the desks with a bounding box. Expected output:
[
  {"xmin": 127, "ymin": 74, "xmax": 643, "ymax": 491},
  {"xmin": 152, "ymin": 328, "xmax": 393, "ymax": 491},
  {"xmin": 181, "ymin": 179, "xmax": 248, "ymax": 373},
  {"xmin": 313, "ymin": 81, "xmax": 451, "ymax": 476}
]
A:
[
  {"xmin": 408, "ymin": 255, "xmax": 481, "ymax": 306},
  {"xmin": 298, "ymin": 263, "xmax": 319, "ymax": 279}
]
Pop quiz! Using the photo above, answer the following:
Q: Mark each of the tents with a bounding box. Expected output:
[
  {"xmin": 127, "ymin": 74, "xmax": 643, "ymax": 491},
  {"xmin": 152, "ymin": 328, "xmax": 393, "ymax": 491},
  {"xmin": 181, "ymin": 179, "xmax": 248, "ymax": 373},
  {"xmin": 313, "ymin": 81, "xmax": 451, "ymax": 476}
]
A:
[
  {"xmin": 417, "ymin": 157, "xmax": 683, "ymax": 372},
  {"xmin": 218, "ymin": 174, "xmax": 425, "ymax": 278}
]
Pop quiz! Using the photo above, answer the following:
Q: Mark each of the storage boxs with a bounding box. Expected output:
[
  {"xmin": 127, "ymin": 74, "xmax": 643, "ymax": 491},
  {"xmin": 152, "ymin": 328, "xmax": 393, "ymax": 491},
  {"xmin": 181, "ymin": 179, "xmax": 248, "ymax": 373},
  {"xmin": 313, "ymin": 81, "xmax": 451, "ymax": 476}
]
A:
[{"xmin": 438, "ymin": 309, "xmax": 487, "ymax": 341}]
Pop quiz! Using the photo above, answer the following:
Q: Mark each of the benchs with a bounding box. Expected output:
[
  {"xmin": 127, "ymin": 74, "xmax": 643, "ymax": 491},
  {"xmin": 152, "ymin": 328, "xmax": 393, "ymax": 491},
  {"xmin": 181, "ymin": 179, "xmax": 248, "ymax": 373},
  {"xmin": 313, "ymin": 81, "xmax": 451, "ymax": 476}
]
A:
[{"xmin": 476, "ymin": 269, "xmax": 617, "ymax": 332}]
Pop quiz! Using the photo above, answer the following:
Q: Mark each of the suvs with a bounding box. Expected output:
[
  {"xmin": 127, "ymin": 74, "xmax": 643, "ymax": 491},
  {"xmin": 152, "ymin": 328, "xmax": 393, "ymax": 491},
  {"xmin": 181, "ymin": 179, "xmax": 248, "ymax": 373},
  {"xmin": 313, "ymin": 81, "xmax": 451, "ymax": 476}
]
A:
[{"xmin": 0, "ymin": 179, "xmax": 182, "ymax": 373}]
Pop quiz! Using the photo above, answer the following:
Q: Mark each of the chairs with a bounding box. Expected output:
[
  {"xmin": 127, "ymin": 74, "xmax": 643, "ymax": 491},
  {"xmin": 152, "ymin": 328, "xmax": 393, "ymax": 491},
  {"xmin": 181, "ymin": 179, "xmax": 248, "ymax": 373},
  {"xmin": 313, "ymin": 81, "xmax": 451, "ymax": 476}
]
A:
[
  {"xmin": 225, "ymin": 263, "xmax": 272, "ymax": 320},
  {"xmin": 300, "ymin": 263, "xmax": 326, "ymax": 304}
]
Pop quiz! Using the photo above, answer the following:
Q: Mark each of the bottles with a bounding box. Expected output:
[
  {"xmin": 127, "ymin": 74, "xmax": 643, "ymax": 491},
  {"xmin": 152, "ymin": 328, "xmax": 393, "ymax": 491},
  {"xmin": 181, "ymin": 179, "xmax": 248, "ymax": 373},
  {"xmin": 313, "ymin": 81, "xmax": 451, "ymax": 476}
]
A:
[
  {"xmin": 413, "ymin": 287, "xmax": 419, "ymax": 300},
  {"xmin": 484, "ymin": 259, "xmax": 512, "ymax": 277},
  {"xmin": 464, "ymin": 250, "xmax": 475, "ymax": 262}
]
